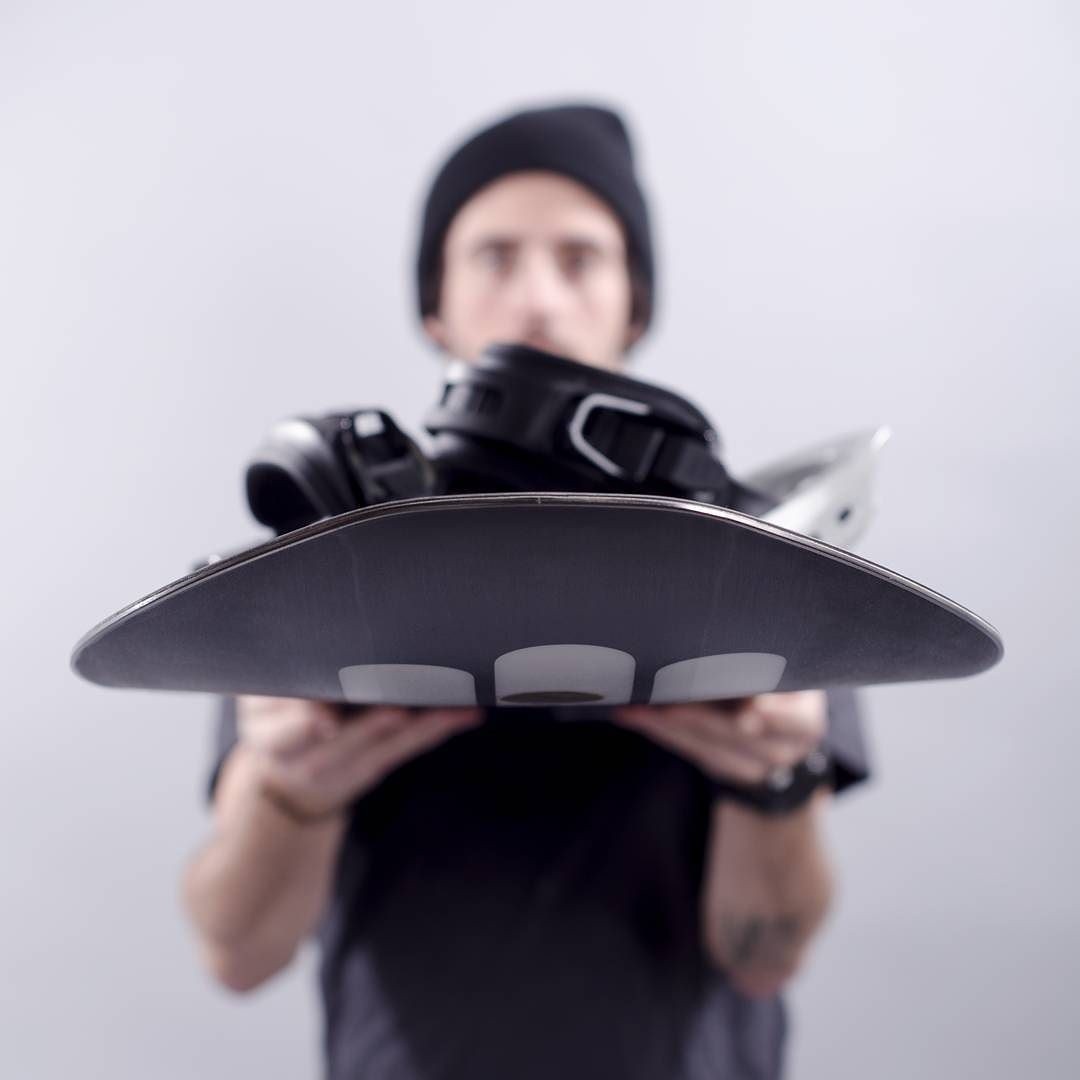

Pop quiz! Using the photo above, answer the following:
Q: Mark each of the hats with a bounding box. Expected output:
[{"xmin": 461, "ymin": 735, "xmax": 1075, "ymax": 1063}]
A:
[{"xmin": 417, "ymin": 106, "xmax": 655, "ymax": 326}]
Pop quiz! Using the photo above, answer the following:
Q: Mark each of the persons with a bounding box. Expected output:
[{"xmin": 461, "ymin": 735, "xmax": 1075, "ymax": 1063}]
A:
[{"xmin": 176, "ymin": 101, "xmax": 841, "ymax": 1080}]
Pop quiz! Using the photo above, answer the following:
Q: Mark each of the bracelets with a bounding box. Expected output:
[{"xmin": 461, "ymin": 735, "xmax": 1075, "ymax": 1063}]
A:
[
  {"xmin": 253, "ymin": 777, "xmax": 344, "ymax": 825},
  {"xmin": 706, "ymin": 756, "xmax": 828, "ymax": 815}
]
